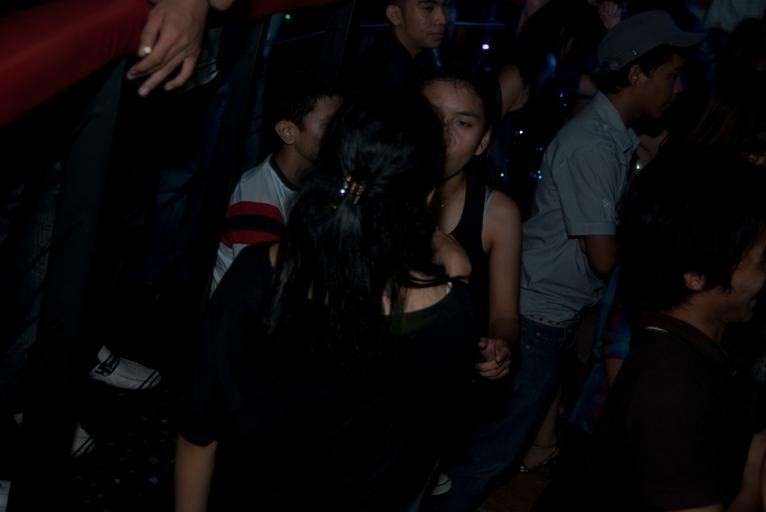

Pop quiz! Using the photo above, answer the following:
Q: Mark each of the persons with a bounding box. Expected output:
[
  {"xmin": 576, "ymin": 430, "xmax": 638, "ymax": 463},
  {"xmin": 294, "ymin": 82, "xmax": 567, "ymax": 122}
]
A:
[{"xmin": 0, "ymin": 0, "xmax": 766, "ymax": 512}]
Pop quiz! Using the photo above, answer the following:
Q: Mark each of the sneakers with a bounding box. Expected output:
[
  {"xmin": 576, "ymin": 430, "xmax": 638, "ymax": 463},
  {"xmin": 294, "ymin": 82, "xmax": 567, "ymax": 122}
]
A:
[{"xmin": 91, "ymin": 346, "xmax": 162, "ymax": 389}]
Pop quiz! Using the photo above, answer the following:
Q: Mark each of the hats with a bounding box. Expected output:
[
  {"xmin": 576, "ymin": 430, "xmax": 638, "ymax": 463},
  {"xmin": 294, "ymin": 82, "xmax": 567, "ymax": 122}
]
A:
[{"xmin": 609, "ymin": 10, "xmax": 696, "ymax": 68}]
